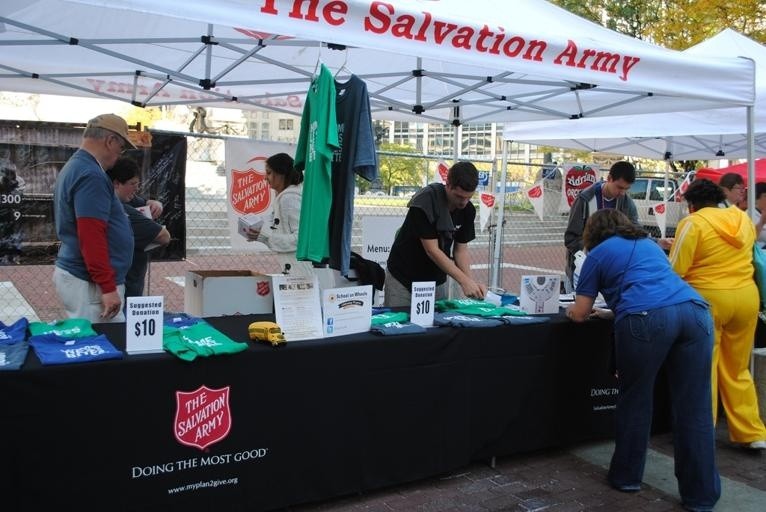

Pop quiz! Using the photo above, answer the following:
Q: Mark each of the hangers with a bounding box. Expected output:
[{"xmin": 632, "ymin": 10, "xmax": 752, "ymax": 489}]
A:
[{"xmin": 311, "ymin": 40, "xmax": 352, "ymax": 84}]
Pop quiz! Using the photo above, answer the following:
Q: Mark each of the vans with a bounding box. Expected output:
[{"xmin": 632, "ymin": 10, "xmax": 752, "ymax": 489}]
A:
[{"xmin": 625, "ymin": 175, "xmax": 688, "ymax": 236}]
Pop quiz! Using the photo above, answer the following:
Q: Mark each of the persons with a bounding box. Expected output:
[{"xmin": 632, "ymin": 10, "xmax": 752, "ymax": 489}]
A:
[
  {"xmin": 244, "ymin": 151, "xmax": 303, "ymax": 255},
  {"xmin": 106, "ymin": 154, "xmax": 171, "ymax": 309},
  {"xmin": 563, "ymin": 204, "xmax": 723, "ymax": 512},
  {"xmin": 563, "ymin": 158, "xmax": 677, "ymax": 291},
  {"xmin": 743, "ymin": 181, "xmax": 765, "ymax": 252},
  {"xmin": 51, "ymin": 111, "xmax": 140, "ymax": 326},
  {"xmin": 662, "ymin": 177, "xmax": 765, "ymax": 454},
  {"xmin": 717, "ymin": 171, "xmax": 765, "ymax": 242},
  {"xmin": 382, "ymin": 160, "xmax": 487, "ymax": 308}
]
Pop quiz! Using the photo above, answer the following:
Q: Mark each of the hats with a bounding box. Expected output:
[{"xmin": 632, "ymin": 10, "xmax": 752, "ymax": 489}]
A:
[{"xmin": 84, "ymin": 113, "xmax": 139, "ymax": 152}]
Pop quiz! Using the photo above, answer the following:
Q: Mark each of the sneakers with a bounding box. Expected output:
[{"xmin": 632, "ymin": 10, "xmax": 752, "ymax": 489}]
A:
[
  {"xmin": 611, "ymin": 481, "xmax": 642, "ymax": 490},
  {"xmin": 740, "ymin": 439, "xmax": 765, "ymax": 449}
]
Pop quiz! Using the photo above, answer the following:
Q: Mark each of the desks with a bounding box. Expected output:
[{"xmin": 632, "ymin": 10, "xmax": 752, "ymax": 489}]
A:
[{"xmin": 0, "ymin": 292, "xmax": 679, "ymax": 512}]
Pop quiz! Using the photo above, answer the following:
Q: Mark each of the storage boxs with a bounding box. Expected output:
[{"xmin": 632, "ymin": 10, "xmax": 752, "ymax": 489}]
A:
[{"xmin": 183, "ymin": 270, "xmax": 275, "ymax": 319}]
